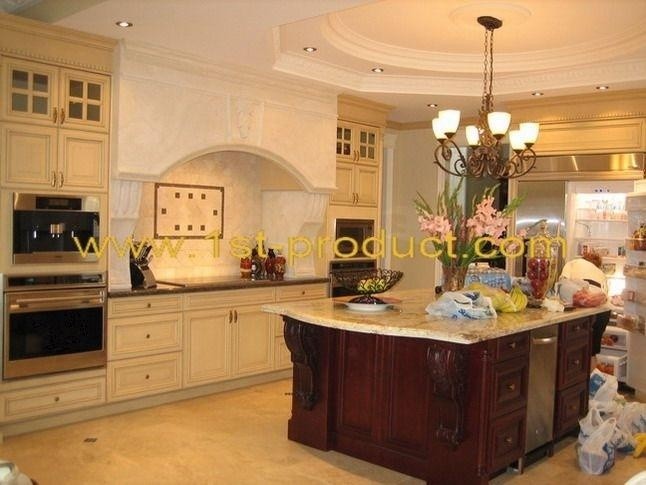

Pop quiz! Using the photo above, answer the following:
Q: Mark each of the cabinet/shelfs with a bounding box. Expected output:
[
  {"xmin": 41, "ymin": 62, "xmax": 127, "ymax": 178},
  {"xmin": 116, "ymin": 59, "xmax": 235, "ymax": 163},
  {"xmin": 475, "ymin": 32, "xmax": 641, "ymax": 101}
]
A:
[
  {"xmin": 554, "ymin": 316, "xmax": 594, "ymax": 443},
  {"xmin": 335, "ymin": 118, "xmax": 380, "ymax": 164},
  {"xmin": 276, "ymin": 284, "xmax": 326, "ymax": 371},
  {"xmin": 185, "ymin": 302, "xmax": 276, "ymax": 383},
  {"xmin": 2, "ymin": 57, "xmax": 111, "ymax": 131},
  {"xmin": 332, "ymin": 158, "xmax": 379, "ymax": 209},
  {"xmin": 483, "ymin": 335, "xmax": 531, "ymax": 468},
  {"xmin": 109, "ymin": 294, "xmax": 183, "ymax": 403},
  {"xmin": 1, "ymin": 125, "xmax": 107, "ymax": 196}
]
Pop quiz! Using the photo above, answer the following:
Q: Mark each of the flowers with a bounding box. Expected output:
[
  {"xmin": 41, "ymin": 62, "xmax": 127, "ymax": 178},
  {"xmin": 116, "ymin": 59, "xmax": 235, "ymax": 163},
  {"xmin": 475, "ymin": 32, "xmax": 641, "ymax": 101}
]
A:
[{"xmin": 410, "ymin": 173, "xmax": 529, "ymax": 267}]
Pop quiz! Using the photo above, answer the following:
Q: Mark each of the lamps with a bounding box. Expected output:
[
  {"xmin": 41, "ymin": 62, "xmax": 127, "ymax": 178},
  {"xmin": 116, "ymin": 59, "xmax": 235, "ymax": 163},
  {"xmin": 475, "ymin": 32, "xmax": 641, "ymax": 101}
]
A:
[{"xmin": 430, "ymin": 17, "xmax": 541, "ymax": 181}]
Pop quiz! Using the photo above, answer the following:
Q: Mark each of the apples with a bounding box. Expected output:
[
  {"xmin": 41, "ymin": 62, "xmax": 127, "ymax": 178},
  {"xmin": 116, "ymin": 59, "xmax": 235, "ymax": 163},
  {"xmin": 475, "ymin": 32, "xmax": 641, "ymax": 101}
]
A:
[{"xmin": 357, "ymin": 279, "xmax": 387, "ymax": 292}]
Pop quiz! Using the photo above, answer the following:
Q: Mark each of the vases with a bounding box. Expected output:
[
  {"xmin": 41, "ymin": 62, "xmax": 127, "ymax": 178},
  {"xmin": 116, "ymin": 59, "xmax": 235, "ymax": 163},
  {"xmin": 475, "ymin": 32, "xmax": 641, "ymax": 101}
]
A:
[{"xmin": 438, "ymin": 264, "xmax": 466, "ymax": 297}]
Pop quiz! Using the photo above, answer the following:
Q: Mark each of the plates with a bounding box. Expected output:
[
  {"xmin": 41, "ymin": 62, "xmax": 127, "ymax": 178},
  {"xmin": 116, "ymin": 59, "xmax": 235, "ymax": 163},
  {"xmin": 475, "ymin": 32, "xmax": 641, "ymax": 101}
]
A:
[{"xmin": 332, "ymin": 296, "xmax": 405, "ymax": 315}]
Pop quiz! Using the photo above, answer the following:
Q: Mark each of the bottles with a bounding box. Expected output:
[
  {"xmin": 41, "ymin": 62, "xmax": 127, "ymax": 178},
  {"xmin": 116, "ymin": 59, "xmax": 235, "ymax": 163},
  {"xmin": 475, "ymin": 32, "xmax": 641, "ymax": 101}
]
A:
[
  {"xmin": 461, "ymin": 266, "xmax": 511, "ymax": 290},
  {"xmin": 585, "ymin": 196, "xmax": 631, "ymax": 220},
  {"xmin": 237, "ymin": 240, "xmax": 292, "ymax": 281}
]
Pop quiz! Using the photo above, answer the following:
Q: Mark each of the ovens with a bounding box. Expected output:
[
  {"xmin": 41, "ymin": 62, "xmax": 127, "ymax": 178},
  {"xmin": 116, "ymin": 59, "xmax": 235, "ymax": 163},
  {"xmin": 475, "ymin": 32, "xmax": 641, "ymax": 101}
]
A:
[{"xmin": 5, "ymin": 193, "xmax": 110, "ymax": 373}]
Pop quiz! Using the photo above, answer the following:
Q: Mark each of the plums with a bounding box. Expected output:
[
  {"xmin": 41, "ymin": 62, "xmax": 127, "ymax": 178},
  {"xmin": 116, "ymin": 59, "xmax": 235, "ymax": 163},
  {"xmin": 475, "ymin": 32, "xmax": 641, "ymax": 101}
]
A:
[{"xmin": 528, "ymin": 259, "xmax": 549, "ymax": 299}]
